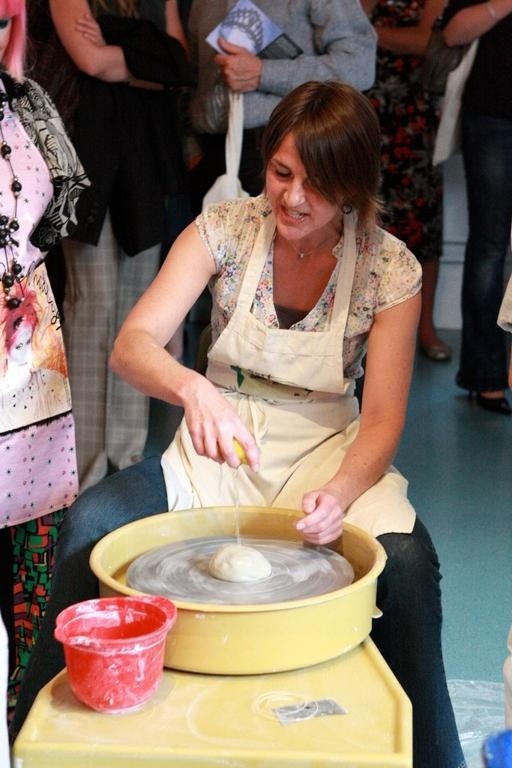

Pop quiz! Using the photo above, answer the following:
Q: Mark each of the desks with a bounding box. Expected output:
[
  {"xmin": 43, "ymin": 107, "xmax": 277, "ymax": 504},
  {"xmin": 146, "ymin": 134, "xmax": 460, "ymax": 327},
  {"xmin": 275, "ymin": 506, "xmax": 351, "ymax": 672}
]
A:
[{"xmin": 10, "ymin": 635, "xmax": 415, "ymax": 768}]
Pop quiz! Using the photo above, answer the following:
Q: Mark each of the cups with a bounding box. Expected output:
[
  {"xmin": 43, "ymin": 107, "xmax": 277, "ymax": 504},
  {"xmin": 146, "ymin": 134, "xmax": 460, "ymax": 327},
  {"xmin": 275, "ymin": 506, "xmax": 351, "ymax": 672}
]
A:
[{"xmin": 50, "ymin": 593, "xmax": 176, "ymax": 715}]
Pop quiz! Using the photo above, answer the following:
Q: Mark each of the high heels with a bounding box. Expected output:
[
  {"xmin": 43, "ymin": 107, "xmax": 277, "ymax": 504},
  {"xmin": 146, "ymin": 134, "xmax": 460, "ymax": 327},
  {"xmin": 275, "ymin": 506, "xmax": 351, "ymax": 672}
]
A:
[{"xmin": 468, "ymin": 388, "xmax": 511, "ymax": 414}]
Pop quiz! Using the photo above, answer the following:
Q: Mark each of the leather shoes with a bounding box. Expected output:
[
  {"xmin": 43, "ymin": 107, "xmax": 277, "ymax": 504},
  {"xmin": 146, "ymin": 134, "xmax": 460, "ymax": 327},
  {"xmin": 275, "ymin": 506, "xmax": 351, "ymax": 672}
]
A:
[{"xmin": 419, "ymin": 342, "xmax": 451, "ymax": 360}]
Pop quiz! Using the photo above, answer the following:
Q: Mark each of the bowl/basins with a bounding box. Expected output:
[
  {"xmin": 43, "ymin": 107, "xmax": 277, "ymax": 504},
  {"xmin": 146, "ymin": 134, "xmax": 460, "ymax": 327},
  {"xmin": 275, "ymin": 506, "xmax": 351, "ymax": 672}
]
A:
[{"xmin": 90, "ymin": 499, "xmax": 389, "ymax": 676}]
